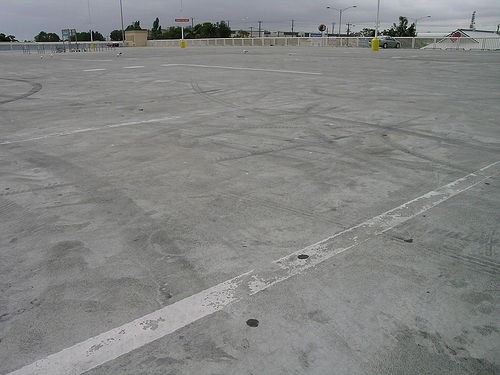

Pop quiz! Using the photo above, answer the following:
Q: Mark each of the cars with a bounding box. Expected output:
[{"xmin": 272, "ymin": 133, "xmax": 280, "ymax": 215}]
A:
[{"xmin": 369, "ymin": 36, "xmax": 401, "ymax": 49}]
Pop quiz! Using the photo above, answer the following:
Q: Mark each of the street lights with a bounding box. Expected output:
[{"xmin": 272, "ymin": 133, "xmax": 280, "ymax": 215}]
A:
[
  {"xmin": 327, "ymin": 6, "xmax": 356, "ymax": 37},
  {"xmin": 402, "ymin": 15, "xmax": 431, "ymax": 37}
]
